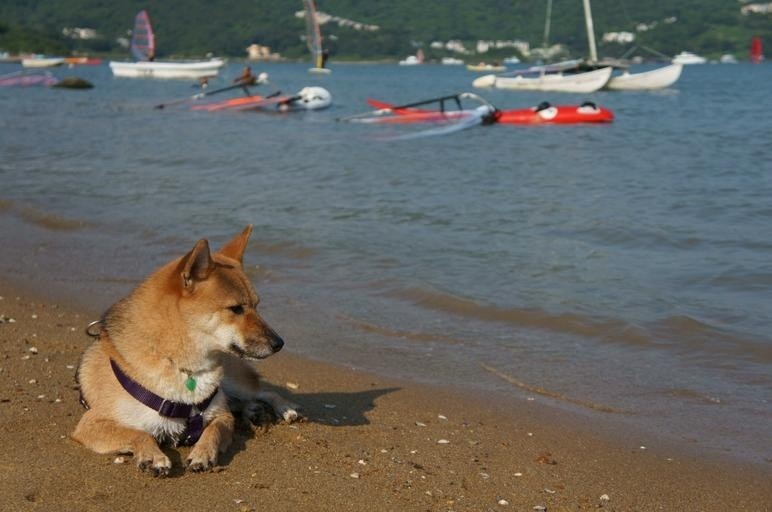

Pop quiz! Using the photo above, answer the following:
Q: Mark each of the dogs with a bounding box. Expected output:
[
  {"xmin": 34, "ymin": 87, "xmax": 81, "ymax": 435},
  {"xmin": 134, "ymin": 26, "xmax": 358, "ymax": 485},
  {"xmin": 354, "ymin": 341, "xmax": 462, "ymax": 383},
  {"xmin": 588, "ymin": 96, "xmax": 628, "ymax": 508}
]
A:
[{"xmin": 68, "ymin": 223, "xmax": 303, "ymax": 479}]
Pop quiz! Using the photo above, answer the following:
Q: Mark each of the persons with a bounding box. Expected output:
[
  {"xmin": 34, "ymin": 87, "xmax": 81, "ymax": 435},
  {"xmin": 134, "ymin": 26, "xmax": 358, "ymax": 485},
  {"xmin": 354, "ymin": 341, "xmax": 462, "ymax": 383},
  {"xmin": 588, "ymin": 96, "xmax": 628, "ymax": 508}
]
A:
[{"xmin": 232, "ymin": 64, "xmax": 257, "ymax": 87}]
